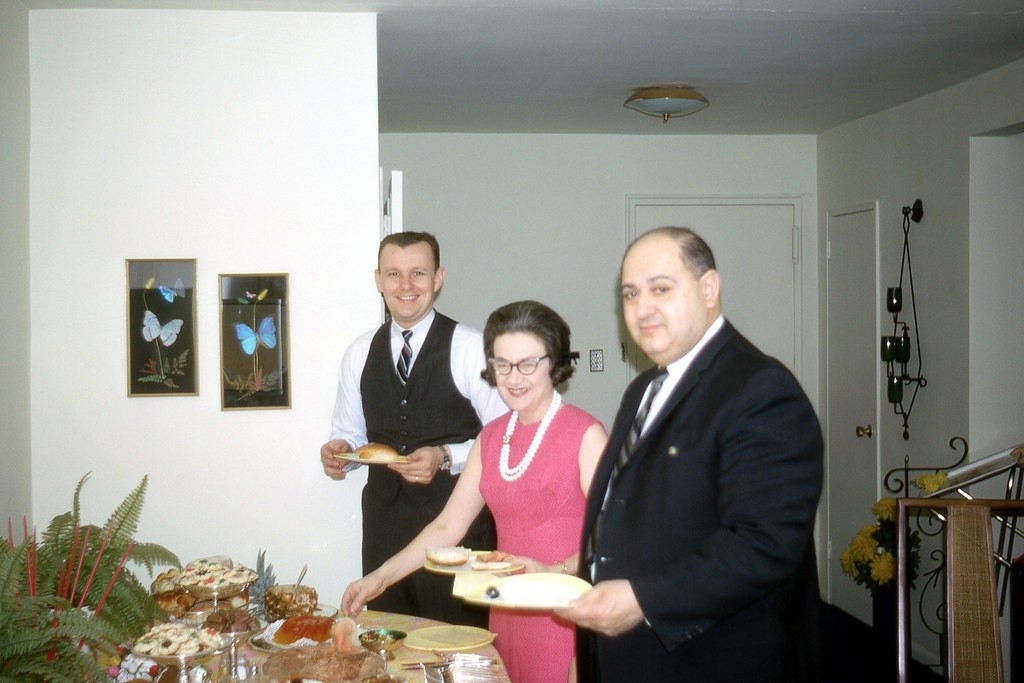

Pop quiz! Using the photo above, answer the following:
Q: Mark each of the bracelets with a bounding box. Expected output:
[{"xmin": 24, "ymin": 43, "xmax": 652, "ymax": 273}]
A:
[{"xmin": 554, "ymin": 561, "xmax": 567, "ymax": 574}]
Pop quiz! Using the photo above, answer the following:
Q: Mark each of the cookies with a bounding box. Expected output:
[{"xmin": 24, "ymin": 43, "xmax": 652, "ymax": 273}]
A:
[
  {"xmin": 177, "ymin": 559, "xmax": 258, "ymax": 588},
  {"xmin": 132, "ymin": 620, "xmax": 227, "ymax": 655}
]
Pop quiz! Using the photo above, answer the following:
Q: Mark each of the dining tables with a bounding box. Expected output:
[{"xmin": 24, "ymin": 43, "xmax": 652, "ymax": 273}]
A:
[{"xmin": 238, "ymin": 607, "xmax": 505, "ymax": 683}]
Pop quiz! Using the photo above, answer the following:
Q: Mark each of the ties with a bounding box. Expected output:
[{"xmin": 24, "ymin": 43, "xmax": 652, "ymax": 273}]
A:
[
  {"xmin": 583, "ymin": 365, "xmax": 669, "ymax": 566},
  {"xmin": 396, "ymin": 330, "xmax": 413, "ymax": 389}
]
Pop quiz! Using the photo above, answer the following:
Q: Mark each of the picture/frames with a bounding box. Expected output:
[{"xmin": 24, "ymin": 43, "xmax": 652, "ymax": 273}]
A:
[
  {"xmin": 218, "ymin": 272, "xmax": 292, "ymax": 411},
  {"xmin": 126, "ymin": 258, "xmax": 199, "ymax": 395}
]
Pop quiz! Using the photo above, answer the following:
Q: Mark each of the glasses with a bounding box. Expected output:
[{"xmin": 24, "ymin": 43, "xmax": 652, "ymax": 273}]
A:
[{"xmin": 488, "ymin": 353, "xmax": 550, "ymax": 376}]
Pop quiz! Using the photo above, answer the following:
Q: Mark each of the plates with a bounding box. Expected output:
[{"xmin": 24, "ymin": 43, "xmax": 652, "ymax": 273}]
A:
[
  {"xmin": 333, "ymin": 452, "xmax": 410, "ymax": 464},
  {"xmin": 247, "ymin": 625, "xmax": 372, "ymax": 655},
  {"xmin": 313, "ymin": 603, "xmax": 337, "ymax": 618},
  {"xmin": 423, "ymin": 551, "xmax": 525, "ymax": 575},
  {"xmin": 463, "ymin": 572, "xmax": 592, "ymax": 610},
  {"xmin": 403, "ymin": 625, "xmax": 496, "ymax": 651}
]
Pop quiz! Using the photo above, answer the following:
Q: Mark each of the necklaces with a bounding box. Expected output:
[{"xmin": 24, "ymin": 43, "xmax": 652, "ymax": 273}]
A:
[{"xmin": 499, "ymin": 387, "xmax": 562, "ymax": 481}]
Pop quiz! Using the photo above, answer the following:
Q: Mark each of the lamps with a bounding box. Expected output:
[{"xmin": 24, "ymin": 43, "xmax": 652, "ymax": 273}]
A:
[
  {"xmin": 882, "ymin": 199, "xmax": 925, "ymax": 442},
  {"xmin": 623, "ymin": 84, "xmax": 709, "ymax": 122}
]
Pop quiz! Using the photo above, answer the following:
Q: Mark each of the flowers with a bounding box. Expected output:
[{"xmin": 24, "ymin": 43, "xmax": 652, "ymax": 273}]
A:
[{"xmin": 841, "ymin": 470, "xmax": 947, "ymax": 593}]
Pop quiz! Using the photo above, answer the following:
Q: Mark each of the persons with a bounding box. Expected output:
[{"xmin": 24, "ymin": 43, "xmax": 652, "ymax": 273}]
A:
[
  {"xmin": 491, "ymin": 226, "xmax": 823, "ymax": 683},
  {"xmin": 320, "ymin": 231, "xmax": 509, "ymax": 631},
  {"xmin": 341, "ymin": 300, "xmax": 610, "ymax": 683}
]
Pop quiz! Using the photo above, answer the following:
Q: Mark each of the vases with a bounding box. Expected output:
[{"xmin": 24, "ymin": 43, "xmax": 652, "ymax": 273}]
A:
[{"xmin": 870, "ymin": 587, "xmax": 914, "ymax": 683}]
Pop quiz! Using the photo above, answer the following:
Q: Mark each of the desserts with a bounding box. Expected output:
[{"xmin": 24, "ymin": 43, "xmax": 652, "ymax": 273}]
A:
[
  {"xmin": 271, "ymin": 614, "xmax": 333, "ymax": 645},
  {"xmin": 203, "ymin": 608, "xmax": 261, "ymax": 633}
]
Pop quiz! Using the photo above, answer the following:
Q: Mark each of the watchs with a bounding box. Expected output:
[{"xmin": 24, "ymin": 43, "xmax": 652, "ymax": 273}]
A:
[{"xmin": 438, "ymin": 445, "xmax": 451, "ymax": 472}]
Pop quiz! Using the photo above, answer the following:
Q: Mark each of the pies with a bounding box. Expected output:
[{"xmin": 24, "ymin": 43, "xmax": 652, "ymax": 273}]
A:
[{"xmin": 261, "ymin": 644, "xmax": 386, "ymax": 683}]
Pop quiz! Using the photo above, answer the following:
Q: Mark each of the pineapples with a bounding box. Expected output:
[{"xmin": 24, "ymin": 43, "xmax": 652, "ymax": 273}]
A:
[{"xmin": 265, "ymin": 583, "xmax": 318, "ymax": 623}]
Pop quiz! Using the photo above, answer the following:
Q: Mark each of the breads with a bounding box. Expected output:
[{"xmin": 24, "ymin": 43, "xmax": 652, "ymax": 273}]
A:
[
  {"xmin": 149, "ymin": 568, "xmax": 249, "ymax": 617},
  {"xmin": 426, "ymin": 545, "xmax": 468, "ymax": 566},
  {"xmin": 355, "ymin": 443, "xmax": 400, "ymax": 460},
  {"xmin": 470, "ymin": 561, "xmax": 512, "ymax": 572}
]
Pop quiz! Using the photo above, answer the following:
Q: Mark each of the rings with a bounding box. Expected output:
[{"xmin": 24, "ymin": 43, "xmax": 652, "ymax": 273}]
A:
[{"xmin": 416, "ymin": 477, "xmax": 418, "ymax": 482}]
